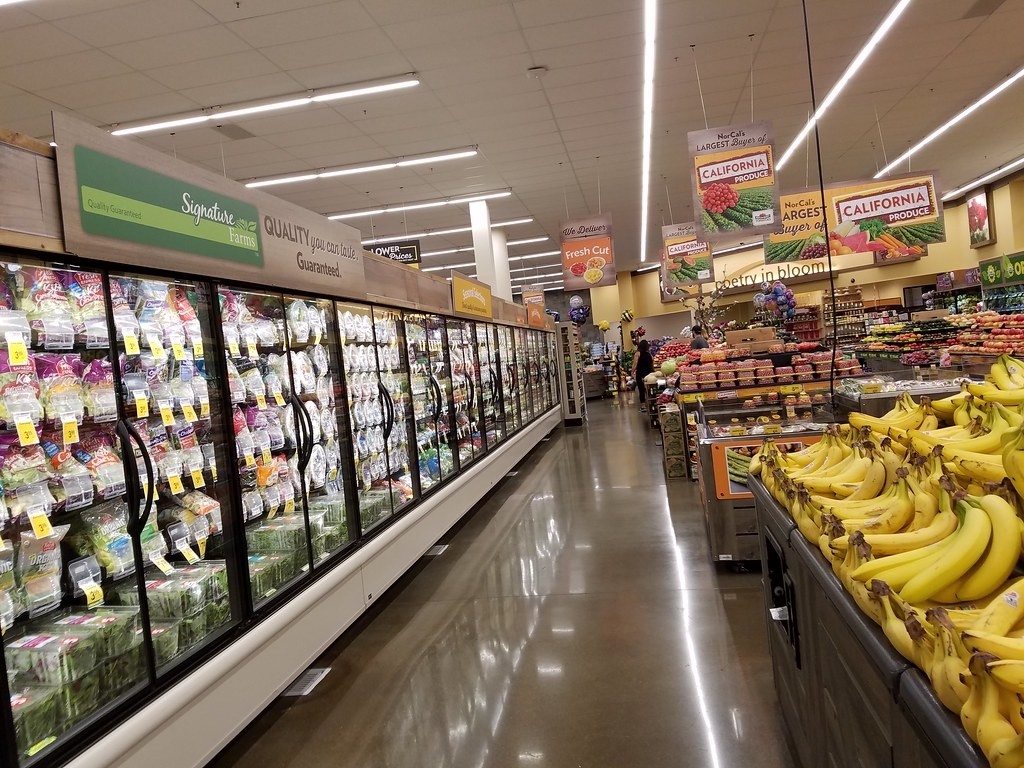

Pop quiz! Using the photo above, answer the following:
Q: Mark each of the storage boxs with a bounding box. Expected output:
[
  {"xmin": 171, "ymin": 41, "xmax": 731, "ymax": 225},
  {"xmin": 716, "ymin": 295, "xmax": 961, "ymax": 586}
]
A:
[{"xmin": 657, "ymin": 403, "xmax": 687, "ymax": 480}]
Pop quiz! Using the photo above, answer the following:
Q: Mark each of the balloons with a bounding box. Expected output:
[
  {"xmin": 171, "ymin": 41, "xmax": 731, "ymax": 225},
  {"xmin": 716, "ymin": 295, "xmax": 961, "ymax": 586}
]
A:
[
  {"xmin": 568, "ymin": 295, "xmax": 591, "ymax": 325},
  {"xmin": 598, "ymin": 321, "xmax": 611, "ymax": 332},
  {"xmin": 753, "ymin": 281, "xmax": 797, "ymax": 319},
  {"xmin": 546, "ymin": 309, "xmax": 560, "ymax": 322},
  {"xmin": 620, "ymin": 309, "xmax": 634, "ymax": 323}
]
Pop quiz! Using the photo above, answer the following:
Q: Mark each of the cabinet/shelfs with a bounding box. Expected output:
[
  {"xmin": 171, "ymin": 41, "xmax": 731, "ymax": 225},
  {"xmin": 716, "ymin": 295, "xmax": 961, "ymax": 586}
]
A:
[
  {"xmin": 556, "ymin": 320, "xmax": 587, "ymax": 419},
  {"xmin": 751, "ymin": 317, "xmax": 820, "ymax": 343},
  {"xmin": 0, "ymin": 246, "xmax": 1024, "ymax": 767},
  {"xmin": 822, "ymin": 291, "xmax": 865, "ymax": 350},
  {"xmin": 643, "ymin": 382, "xmax": 666, "ymax": 430},
  {"xmin": 599, "ymin": 360, "xmax": 617, "ymax": 397}
]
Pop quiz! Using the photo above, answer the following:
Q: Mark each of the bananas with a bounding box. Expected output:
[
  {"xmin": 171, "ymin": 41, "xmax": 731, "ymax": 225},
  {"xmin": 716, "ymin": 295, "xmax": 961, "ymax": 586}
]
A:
[{"xmin": 748, "ymin": 359, "xmax": 1024, "ymax": 768}]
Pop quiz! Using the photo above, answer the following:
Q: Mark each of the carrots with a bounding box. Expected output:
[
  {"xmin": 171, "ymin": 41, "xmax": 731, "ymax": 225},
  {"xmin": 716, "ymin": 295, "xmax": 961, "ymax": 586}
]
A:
[{"xmin": 874, "ymin": 234, "xmax": 906, "ymax": 249}]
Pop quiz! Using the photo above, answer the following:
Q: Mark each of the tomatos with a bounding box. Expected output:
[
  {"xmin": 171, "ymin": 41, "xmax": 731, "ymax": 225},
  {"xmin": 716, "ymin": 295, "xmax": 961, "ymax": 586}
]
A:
[{"xmin": 702, "ymin": 183, "xmax": 739, "ymax": 213}]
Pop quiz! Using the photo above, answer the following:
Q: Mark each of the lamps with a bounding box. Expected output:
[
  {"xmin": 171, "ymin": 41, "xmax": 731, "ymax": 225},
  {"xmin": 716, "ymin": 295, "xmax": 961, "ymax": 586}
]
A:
[
  {"xmin": 635, "ymin": 240, "xmax": 764, "ymax": 273},
  {"xmin": 420, "ymin": 234, "xmax": 549, "ymax": 257},
  {"xmin": 871, "ymin": 69, "xmax": 1024, "ymax": 179},
  {"xmin": 775, "ymin": 0, "xmax": 910, "ymax": 175},
  {"xmin": 941, "ymin": 158, "xmax": 1024, "ymax": 201},
  {"xmin": 236, "ymin": 144, "xmax": 479, "ymax": 187},
  {"xmin": 421, "ymin": 250, "xmax": 565, "ymax": 296},
  {"xmin": 322, "ymin": 187, "xmax": 512, "ymax": 219},
  {"xmin": 35, "ymin": 72, "xmax": 421, "ymax": 145},
  {"xmin": 361, "ymin": 215, "xmax": 534, "ymax": 246}
]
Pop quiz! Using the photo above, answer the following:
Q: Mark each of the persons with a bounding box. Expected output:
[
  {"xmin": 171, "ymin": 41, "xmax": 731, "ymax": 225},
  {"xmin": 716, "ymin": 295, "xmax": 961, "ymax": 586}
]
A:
[
  {"xmin": 690, "ymin": 325, "xmax": 709, "ymax": 349},
  {"xmin": 631, "ymin": 340, "xmax": 654, "ymax": 411}
]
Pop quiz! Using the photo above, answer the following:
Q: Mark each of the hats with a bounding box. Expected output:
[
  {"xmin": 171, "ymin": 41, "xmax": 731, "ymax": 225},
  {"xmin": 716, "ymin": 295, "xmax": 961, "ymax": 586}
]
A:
[{"xmin": 690, "ymin": 325, "xmax": 701, "ymax": 333}]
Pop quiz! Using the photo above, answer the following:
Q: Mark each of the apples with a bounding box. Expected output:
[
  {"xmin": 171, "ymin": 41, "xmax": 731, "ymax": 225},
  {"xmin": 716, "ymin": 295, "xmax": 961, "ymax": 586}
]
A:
[{"xmin": 950, "ymin": 313, "xmax": 1024, "ymax": 357}]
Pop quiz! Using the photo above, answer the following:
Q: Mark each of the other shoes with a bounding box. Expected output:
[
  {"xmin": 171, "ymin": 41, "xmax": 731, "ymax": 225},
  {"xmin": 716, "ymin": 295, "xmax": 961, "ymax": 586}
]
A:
[{"xmin": 639, "ymin": 407, "xmax": 647, "ymax": 412}]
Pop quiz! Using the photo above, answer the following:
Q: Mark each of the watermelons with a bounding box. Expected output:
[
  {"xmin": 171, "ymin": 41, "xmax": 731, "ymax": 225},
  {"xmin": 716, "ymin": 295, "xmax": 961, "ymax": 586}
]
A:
[
  {"xmin": 673, "ymin": 257, "xmax": 682, "ymax": 262},
  {"xmin": 667, "ymin": 263, "xmax": 682, "ymax": 273},
  {"xmin": 681, "ymin": 257, "xmax": 696, "ymax": 268}
]
